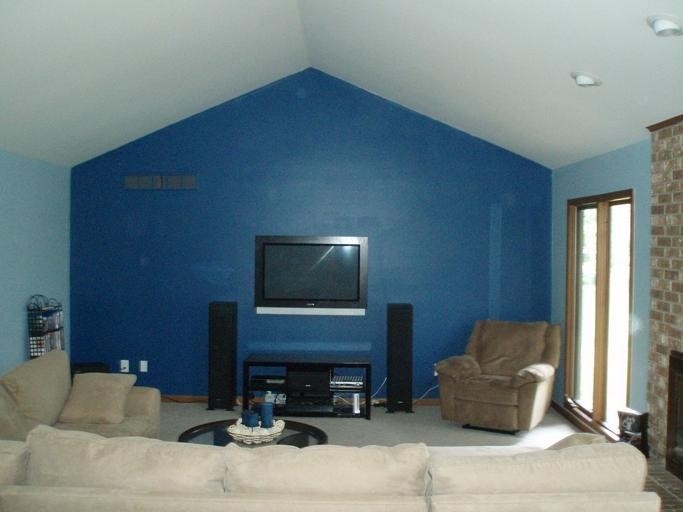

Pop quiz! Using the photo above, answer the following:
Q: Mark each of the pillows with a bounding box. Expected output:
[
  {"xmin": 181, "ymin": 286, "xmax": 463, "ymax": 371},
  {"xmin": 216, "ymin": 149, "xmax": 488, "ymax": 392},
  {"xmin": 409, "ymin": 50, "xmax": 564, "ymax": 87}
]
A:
[
  {"xmin": 58, "ymin": 374, "xmax": 134, "ymax": 424},
  {"xmin": 546, "ymin": 432, "xmax": 607, "ymax": 450}
]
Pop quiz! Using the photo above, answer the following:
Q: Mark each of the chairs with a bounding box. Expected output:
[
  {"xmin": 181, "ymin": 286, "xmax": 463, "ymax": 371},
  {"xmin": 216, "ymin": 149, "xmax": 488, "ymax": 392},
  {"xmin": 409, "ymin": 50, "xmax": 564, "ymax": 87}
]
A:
[{"xmin": 433, "ymin": 320, "xmax": 560, "ymax": 435}]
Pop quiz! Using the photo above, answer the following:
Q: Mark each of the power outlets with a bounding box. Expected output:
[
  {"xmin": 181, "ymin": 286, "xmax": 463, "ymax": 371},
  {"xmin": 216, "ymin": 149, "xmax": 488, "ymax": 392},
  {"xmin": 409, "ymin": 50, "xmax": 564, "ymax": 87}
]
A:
[{"xmin": 120, "ymin": 360, "xmax": 129, "ymax": 372}]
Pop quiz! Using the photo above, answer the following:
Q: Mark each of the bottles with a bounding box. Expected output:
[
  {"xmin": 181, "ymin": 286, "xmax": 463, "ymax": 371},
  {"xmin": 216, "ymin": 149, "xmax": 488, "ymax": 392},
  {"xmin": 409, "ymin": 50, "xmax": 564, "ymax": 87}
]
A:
[{"xmin": 259, "ymin": 402, "xmax": 274, "ymax": 428}]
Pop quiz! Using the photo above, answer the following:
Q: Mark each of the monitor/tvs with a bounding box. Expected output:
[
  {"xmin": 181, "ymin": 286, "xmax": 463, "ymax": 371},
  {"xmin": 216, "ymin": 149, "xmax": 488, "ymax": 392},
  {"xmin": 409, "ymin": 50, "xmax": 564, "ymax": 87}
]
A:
[{"xmin": 254, "ymin": 234, "xmax": 369, "ymax": 316}]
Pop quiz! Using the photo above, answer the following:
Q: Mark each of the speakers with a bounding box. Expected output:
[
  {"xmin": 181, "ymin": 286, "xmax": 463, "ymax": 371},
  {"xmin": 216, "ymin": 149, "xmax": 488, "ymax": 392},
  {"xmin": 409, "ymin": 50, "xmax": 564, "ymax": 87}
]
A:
[
  {"xmin": 386, "ymin": 302, "xmax": 413, "ymax": 413},
  {"xmin": 208, "ymin": 300, "xmax": 238, "ymax": 409}
]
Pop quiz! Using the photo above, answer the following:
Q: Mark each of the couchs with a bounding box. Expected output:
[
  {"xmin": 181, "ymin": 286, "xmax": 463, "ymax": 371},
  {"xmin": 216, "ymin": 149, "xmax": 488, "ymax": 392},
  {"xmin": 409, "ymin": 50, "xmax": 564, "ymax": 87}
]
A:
[
  {"xmin": 0, "ymin": 423, "xmax": 662, "ymax": 511},
  {"xmin": 0, "ymin": 350, "xmax": 160, "ymax": 440}
]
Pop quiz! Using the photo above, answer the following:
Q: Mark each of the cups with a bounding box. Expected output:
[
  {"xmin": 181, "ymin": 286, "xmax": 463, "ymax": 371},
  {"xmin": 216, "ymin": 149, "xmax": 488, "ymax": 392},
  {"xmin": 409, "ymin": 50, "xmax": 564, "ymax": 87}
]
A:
[{"xmin": 240, "ymin": 409, "xmax": 259, "ymax": 427}]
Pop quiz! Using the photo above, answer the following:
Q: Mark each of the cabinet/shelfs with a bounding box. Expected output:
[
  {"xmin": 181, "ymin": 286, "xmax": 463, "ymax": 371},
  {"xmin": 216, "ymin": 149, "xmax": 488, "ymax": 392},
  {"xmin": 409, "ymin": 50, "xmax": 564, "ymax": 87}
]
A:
[
  {"xmin": 26, "ymin": 298, "xmax": 65, "ymax": 358},
  {"xmin": 243, "ymin": 350, "xmax": 371, "ymax": 421}
]
